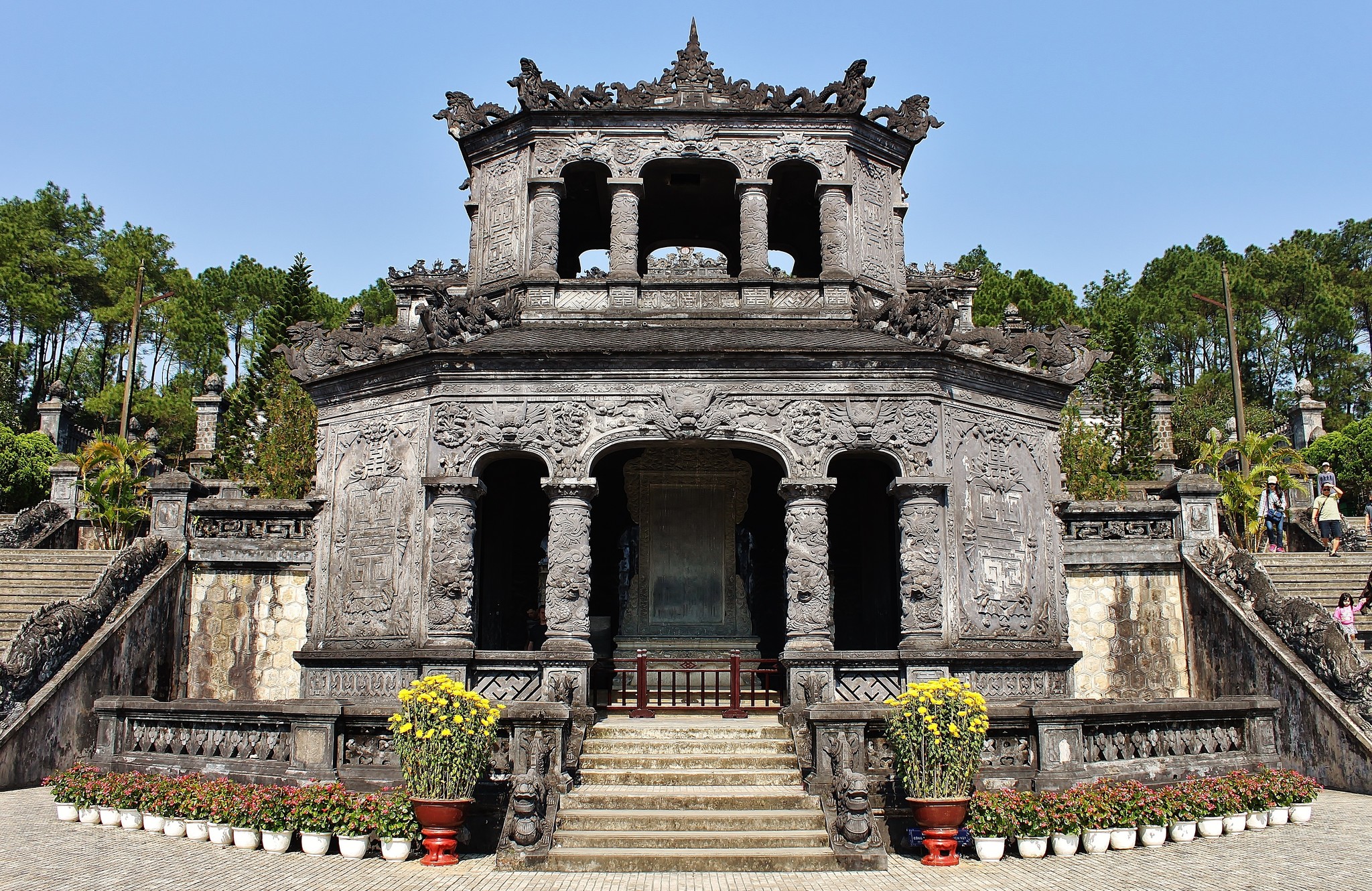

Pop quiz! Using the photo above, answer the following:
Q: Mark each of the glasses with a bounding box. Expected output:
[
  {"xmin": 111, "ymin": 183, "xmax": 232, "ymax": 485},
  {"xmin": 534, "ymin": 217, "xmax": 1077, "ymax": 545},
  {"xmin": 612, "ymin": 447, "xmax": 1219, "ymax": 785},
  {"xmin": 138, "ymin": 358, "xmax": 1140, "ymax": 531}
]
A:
[
  {"xmin": 1269, "ymin": 483, "xmax": 1276, "ymax": 485},
  {"xmin": 1323, "ymin": 488, "xmax": 1330, "ymax": 491}
]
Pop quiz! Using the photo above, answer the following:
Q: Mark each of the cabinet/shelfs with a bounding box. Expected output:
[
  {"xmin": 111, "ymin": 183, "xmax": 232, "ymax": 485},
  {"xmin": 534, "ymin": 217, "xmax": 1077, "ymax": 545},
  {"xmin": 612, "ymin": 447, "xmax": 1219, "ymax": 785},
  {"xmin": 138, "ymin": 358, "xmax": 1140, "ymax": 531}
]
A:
[{"xmin": 297, "ymin": 829, "xmax": 332, "ymax": 856}]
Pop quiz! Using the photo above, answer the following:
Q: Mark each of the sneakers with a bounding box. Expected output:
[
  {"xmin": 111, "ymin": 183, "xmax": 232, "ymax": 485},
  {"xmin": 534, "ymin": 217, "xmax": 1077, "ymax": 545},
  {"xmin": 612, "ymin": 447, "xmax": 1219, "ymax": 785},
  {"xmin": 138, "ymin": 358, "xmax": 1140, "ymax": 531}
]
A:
[
  {"xmin": 1276, "ymin": 547, "xmax": 1284, "ymax": 553},
  {"xmin": 1269, "ymin": 544, "xmax": 1276, "ymax": 552}
]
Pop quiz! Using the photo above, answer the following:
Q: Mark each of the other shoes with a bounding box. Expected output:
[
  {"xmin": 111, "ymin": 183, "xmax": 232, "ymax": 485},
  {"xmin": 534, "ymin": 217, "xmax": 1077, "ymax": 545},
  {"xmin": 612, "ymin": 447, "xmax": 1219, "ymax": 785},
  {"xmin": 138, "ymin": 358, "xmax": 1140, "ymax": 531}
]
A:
[
  {"xmin": 1324, "ymin": 546, "xmax": 1329, "ymax": 552},
  {"xmin": 1329, "ymin": 553, "xmax": 1341, "ymax": 558}
]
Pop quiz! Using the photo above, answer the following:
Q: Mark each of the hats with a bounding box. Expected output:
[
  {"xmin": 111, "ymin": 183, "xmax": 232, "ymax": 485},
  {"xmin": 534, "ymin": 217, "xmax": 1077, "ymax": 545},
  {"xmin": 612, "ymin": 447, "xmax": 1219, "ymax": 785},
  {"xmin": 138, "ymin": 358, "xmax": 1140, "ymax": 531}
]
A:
[
  {"xmin": 1267, "ymin": 475, "xmax": 1277, "ymax": 484},
  {"xmin": 1322, "ymin": 462, "xmax": 1330, "ymax": 466}
]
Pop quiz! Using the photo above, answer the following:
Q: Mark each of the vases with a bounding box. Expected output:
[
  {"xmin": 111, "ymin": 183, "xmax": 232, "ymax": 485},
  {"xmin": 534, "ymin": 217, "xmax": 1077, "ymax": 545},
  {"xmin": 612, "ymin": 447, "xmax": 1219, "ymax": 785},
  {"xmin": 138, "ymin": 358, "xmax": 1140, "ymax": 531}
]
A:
[
  {"xmin": 99, "ymin": 806, "xmax": 120, "ymax": 827},
  {"xmin": 207, "ymin": 820, "xmax": 233, "ymax": 847},
  {"xmin": 1288, "ymin": 802, "xmax": 1314, "ymax": 824},
  {"xmin": 973, "ymin": 809, "xmax": 1268, "ymax": 862},
  {"xmin": 904, "ymin": 796, "xmax": 972, "ymax": 829},
  {"xmin": 55, "ymin": 801, "xmax": 80, "ymax": 822},
  {"xmin": 378, "ymin": 836, "xmax": 412, "ymax": 863},
  {"xmin": 335, "ymin": 833, "xmax": 370, "ymax": 861},
  {"xmin": 141, "ymin": 810, "xmax": 164, "ymax": 833},
  {"xmin": 232, "ymin": 826, "xmax": 260, "ymax": 850},
  {"xmin": 117, "ymin": 807, "xmax": 143, "ymax": 831},
  {"xmin": 260, "ymin": 828, "xmax": 295, "ymax": 856},
  {"xmin": 183, "ymin": 819, "xmax": 209, "ymax": 842},
  {"xmin": 163, "ymin": 815, "xmax": 186, "ymax": 839},
  {"xmin": 79, "ymin": 804, "xmax": 100, "ymax": 824},
  {"xmin": 1268, "ymin": 806, "xmax": 1289, "ymax": 827},
  {"xmin": 409, "ymin": 796, "xmax": 475, "ymax": 830}
]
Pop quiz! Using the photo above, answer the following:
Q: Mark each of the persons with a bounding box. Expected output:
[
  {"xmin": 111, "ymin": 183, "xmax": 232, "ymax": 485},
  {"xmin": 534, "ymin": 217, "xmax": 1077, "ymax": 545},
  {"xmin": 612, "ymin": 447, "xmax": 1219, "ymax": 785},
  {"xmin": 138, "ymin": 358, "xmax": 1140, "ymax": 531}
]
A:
[
  {"xmin": 1333, "ymin": 593, "xmax": 1366, "ymax": 643},
  {"xmin": 1311, "ymin": 482, "xmax": 1343, "ymax": 557},
  {"xmin": 1257, "ymin": 475, "xmax": 1286, "ymax": 552},
  {"xmin": 1363, "ymin": 488, "xmax": 1372, "ymax": 536},
  {"xmin": 1317, "ymin": 462, "xmax": 1336, "ymax": 496},
  {"xmin": 1359, "ymin": 570, "xmax": 1372, "ymax": 650}
]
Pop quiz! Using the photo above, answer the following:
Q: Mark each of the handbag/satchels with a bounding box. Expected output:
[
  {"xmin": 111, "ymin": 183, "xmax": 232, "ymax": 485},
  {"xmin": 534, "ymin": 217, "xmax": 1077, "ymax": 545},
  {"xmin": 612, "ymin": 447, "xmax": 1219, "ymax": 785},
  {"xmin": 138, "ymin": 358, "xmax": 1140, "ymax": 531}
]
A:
[
  {"xmin": 1317, "ymin": 517, "xmax": 1320, "ymax": 529},
  {"xmin": 1267, "ymin": 509, "xmax": 1282, "ymax": 522}
]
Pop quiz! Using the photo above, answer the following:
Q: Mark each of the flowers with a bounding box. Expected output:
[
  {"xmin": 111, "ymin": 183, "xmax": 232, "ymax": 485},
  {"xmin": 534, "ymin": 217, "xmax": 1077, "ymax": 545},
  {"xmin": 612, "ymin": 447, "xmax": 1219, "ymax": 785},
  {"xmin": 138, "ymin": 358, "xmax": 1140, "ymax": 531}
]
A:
[
  {"xmin": 40, "ymin": 671, "xmax": 508, "ymax": 847},
  {"xmin": 881, "ymin": 674, "xmax": 1325, "ymax": 837}
]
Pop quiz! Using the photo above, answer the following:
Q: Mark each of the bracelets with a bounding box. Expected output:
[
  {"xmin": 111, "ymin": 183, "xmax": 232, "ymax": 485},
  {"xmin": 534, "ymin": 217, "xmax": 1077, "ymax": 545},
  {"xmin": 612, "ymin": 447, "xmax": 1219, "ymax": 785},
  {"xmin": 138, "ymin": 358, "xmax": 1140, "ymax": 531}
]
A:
[
  {"xmin": 1332, "ymin": 485, "xmax": 1336, "ymax": 488},
  {"xmin": 1311, "ymin": 518, "xmax": 1315, "ymax": 519}
]
